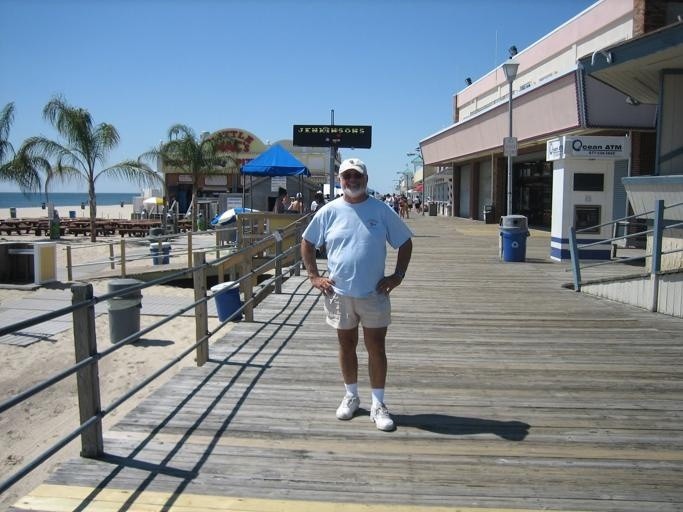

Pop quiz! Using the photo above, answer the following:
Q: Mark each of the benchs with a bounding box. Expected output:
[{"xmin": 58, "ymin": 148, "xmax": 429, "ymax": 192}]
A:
[{"xmin": 0, "ymin": 213, "xmax": 200, "ymax": 238}]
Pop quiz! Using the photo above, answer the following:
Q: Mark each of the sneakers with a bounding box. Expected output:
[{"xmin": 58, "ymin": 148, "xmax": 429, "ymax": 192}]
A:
[
  {"xmin": 335, "ymin": 389, "xmax": 362, "ymax": 420},
  {"xmin": 368, "ymin": 400, "xmax": 395, "ymax": 432}
]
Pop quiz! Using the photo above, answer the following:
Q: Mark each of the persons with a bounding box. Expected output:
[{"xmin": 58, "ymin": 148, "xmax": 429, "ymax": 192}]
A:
[
  {"xmin": 299, "ymin": 156, "xmax": 412, "ymax": 431},
  {"xmin": 413, "ymin": 196, "xmax": 421, "ymax": 214},
  {"xmin": 140, "ymin": 208, "xmax": 147, "ymax": 219},
  {"xmin": 310, "ymin": 190, "xmax": 329, "ymax": 211},
  {"xmin": 286, "ymin": 192, "xmax": 305, "ymax": 212},
  {"xmin": 380, "ymin": 193, "xmax": 413, "ymax": 220},
  {"xmin": 272, "ymin": 187, "xmax": 287, "ymax": 214}
]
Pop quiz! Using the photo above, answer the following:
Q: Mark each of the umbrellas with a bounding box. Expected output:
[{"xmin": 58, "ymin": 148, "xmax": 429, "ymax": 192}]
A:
[
  {"xmin": 142, "ymin": 196, "xmax": 163, "ymax": 214},
  {"xmin": 209, "ymin": 206, "xmax": 258, "ymax": 225}
]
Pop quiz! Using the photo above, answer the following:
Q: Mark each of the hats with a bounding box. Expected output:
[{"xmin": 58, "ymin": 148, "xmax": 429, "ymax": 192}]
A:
[{"xmin": 339, "ymin": 158, "xmax": 368, "ymax": 177}]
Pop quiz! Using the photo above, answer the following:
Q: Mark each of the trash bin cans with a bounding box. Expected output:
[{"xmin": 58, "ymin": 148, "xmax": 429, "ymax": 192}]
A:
[
  {"xmin": 498, "ymin": 215, "xmax": 530, "ymax": 262},
  {"xmin": 210, "ymin": 280, "xmax": 243, "ymax": 321},
  {"xmin": 70, "ymin": 211, "xmax": 75, "ymax": 218},
  {"xmin": 428, "ymin": 202, "xmax": 437, "ymax": 216},
  {"xmin": 151, "ymin": 242, "xmax": 172, "ymax": 265},
  {"xmin": 10, "ymin": 207, "xmax": 16, "ymax": 219},
  {"xmin": 107, "ymin": 278, "xmax": 143, "ymax": 343},
  {"xmin": 483, "ymin": 205, "xmax": 496, "ymax": 224}
]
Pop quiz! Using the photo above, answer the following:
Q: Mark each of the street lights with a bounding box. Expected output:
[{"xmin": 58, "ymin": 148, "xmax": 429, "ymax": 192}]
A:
[
  {"xmin": 392, "ymin": 151, "xmax": 426, "ymax": 216},
  {"xmin": 498, "ymin": 57, "xmax": 519, "ymax": 216}
]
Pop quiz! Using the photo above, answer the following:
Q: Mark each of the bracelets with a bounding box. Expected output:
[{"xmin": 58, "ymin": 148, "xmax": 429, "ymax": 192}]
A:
[{"xmin": 394, "ymin": 270, "xmax": 405, "ymax": 279}]
